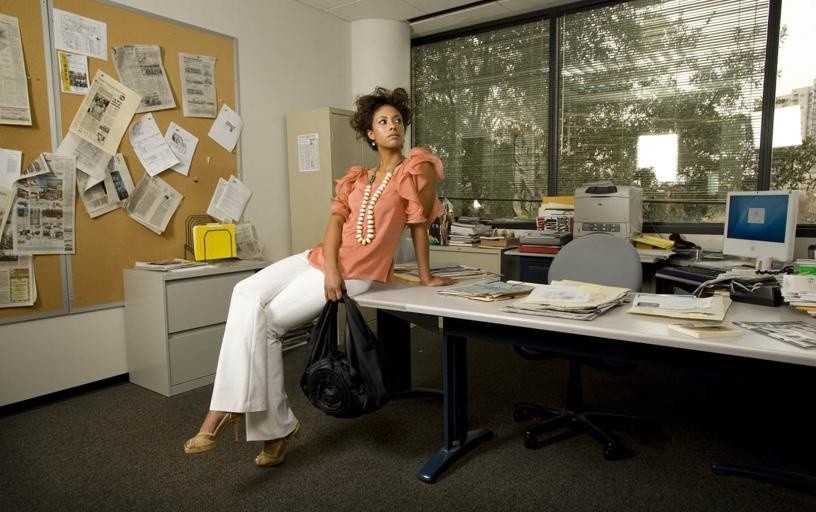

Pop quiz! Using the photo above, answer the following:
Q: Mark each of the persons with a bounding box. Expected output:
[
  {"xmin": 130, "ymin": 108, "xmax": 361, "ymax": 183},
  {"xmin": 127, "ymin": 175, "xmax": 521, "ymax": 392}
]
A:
[{"xmin": 184, "ymin": 86, "xmax": 456, "ymax": 468}]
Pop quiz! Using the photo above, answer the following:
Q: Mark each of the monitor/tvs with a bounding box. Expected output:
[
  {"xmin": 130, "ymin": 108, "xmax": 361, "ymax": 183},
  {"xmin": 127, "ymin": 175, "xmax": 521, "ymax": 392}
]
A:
[{"xmin": 721, "ymin": 190, "xmax": 799, "ymax": 273}]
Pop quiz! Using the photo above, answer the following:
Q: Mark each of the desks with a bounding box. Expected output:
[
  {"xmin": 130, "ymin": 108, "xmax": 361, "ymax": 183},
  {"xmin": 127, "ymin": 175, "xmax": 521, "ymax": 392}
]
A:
[{"xmin": 357, "ymin": 244, "xmax": 816, "ymax": 485}]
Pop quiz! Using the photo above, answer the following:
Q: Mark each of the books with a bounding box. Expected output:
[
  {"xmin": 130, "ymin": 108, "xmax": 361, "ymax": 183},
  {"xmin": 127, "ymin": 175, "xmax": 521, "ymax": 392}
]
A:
[
  {"xmin": 633, "ymin": 235, "xmax": 675, "ymax": 264},
  {"xmin": 134, "ymin": 258, "xmax": 207, "ymax": 271},
  {"xmin": 448, "ymin": 216, "xmax": 511, "ymax": 247},
  {"xmin": 781, "ymin": 258, "xmax": 816, "ymax": 318}
]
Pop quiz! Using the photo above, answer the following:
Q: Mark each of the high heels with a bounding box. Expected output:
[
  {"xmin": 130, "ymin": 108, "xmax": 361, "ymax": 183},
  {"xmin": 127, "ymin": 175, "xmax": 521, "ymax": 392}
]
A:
[
  {"xmin": 184, "ymin": 412, "xmax": 242, "ymax": 454},
  {"xmin": 254, "ymin": 421, "xmax": 303, "ymax": 467}
]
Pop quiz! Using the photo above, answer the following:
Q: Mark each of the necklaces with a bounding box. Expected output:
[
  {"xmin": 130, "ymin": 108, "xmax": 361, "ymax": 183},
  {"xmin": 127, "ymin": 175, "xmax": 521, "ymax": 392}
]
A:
[{"xmin": 356, "ymin": 157, "xmax": 405, "ymax": 244}]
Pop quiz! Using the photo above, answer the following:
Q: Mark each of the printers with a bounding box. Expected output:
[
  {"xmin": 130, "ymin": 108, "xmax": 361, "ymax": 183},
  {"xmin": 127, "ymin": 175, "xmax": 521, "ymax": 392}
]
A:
[{"xmin": 571, "ymin": 183, "xmax": 643, "ymax": 239}]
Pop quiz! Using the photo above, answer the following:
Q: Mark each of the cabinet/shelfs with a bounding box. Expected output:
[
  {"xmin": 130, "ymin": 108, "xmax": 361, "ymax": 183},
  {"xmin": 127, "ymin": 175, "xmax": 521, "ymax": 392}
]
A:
[
  {"xmin": 121, "ymin": 261, "xmax": 271, "ymax": 400},
  {"xmin": 286, "ymin": 106, "xmax": 382, "ymax": 255}
]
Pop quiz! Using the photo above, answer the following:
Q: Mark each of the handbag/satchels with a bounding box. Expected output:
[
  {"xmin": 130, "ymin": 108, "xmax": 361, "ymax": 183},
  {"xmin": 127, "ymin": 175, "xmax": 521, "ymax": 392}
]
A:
[{"xmin": 300, "ymin": 295, "xmax": 389, "ymax": 418}]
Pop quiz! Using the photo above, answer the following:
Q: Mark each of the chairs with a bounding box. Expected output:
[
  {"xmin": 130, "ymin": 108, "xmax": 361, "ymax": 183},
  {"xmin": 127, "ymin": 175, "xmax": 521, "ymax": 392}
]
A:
[{"xmin": 512, "ymin": 234, "xmax": 648, "ymax": 463}]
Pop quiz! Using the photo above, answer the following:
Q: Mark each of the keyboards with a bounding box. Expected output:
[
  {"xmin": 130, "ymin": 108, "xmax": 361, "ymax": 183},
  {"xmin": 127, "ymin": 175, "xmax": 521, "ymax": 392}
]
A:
[{"xmin": 658, "ymin": 264, "xmax": 730, "ymax": 283}]
